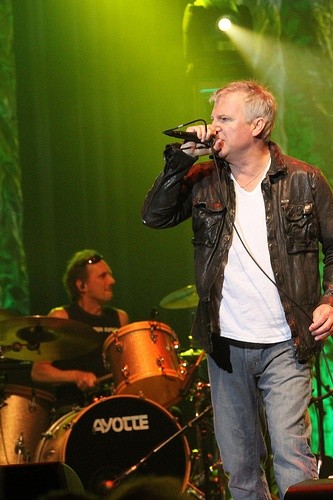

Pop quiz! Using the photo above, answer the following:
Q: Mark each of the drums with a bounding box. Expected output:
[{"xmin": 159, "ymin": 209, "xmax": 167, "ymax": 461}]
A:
[
  {"xmin": 195, "ymin": 382, "xmax": 273, "ymax": 500},
  {"xmin": 0, "ymin": 383, "xmax": 56, "ymax": 465},
  {"xmin": 103, "ymin": 321, "xmax": 187, "ymax": 407},
  {"xmin": 35, "ymin": 394, "xmax": 190, "ymax": 500}
]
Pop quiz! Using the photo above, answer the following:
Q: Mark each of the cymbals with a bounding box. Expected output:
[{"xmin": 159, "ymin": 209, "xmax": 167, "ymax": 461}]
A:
[
  {"xmin": 160, "ymin": 284, "xmax": 203, "ymax": 309},
  {"xmin": 0, "ymin": 315, "xmax": 86, "ymax": 361}
]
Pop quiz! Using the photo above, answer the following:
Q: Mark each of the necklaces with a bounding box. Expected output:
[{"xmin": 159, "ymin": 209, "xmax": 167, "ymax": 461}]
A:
[{"xmin": 231, "ymin": 152, "xmax": 270, "ymax": 192}]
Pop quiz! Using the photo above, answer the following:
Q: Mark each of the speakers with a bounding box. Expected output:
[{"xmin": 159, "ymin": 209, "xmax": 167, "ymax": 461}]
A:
[
  {"xmin": 284, "ymin": 479, "xmax": 333, "ymax": 500},
  {"xmin": 0, "ymin": 461, "xmax": 85, "ymax": 500}
]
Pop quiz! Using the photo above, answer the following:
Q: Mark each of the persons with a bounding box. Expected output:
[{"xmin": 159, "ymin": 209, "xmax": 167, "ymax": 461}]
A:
[
  {"xmin": 31, "ymin": 250, "xmax": 129, "ymax": 418},
  {"xmin": 141, "ymin": 82, "xmax": 333, "ymax": 500}
]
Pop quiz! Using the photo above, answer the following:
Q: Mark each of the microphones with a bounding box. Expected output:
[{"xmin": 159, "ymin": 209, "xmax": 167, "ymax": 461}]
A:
[
  {"xmin": 163, "ymin": 130, "xmax": 217, "ymax": 148},
  {"xmin": 98, "ymin": 480, "xmax": 114, "ymax": 493}
]
